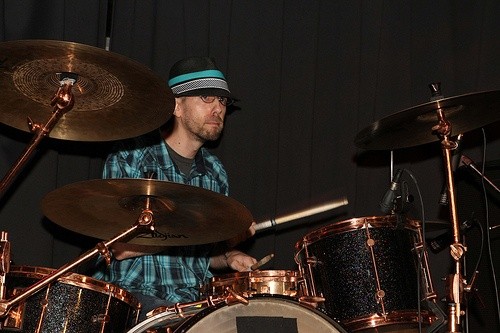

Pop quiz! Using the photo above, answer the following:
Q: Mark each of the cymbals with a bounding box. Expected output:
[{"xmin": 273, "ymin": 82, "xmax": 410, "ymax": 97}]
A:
[
  {"xmin": 40, "ymin": 178, "xmax": 253, "ymax": 247},
  {"xmin": 354, "ymin": 88, "xmax": 500, "ymax": 152},
  {"xmin": 1, "ymin": 38, "xmax": 175, "ymax": 142}
]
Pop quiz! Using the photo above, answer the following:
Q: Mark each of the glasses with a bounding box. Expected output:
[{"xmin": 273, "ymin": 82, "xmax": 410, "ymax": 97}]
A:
[{"xmin": 200, "ymin": 96, "xmax": 234, "ymax": 106}]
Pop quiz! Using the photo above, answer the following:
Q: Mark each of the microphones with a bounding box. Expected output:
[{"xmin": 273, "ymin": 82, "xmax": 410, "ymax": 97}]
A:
[
  {"xmin": 429, "ymin": 218, "xmax": 477, "ymax": 250},
  {"xmin": 378, "ymin": 167, "xmax": 404, "ymax": 213},
  {"xmin": 439, "ymin": 134, "xmax": 461, "ymax": 207}
]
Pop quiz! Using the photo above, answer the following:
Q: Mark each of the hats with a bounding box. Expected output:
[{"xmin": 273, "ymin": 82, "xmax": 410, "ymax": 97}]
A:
[{"xmin": 168, "ymin": 56, "xmax": 232, "ymax": 96}]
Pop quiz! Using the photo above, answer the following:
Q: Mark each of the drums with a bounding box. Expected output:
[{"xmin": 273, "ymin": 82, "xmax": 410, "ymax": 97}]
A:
[
  {"xmin": 0, "ymin": 264, "xmax": 135, "ymax": 333},
  {"xmin": 127, "ymin": 294, "xmax": 348, "ymax": 333},
  {"xmin": 202, "ymin": 268, "xmax": 304, "ymax": 300},
  {"xmin": 292, "ymin": 213, "xmax": 432, "ymax": 332}
]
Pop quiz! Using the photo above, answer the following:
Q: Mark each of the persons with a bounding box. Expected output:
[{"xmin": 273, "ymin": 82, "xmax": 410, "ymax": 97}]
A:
[{"xmin": 92, "ymin": 56, "xmax": 258, "ymax": 313}]
